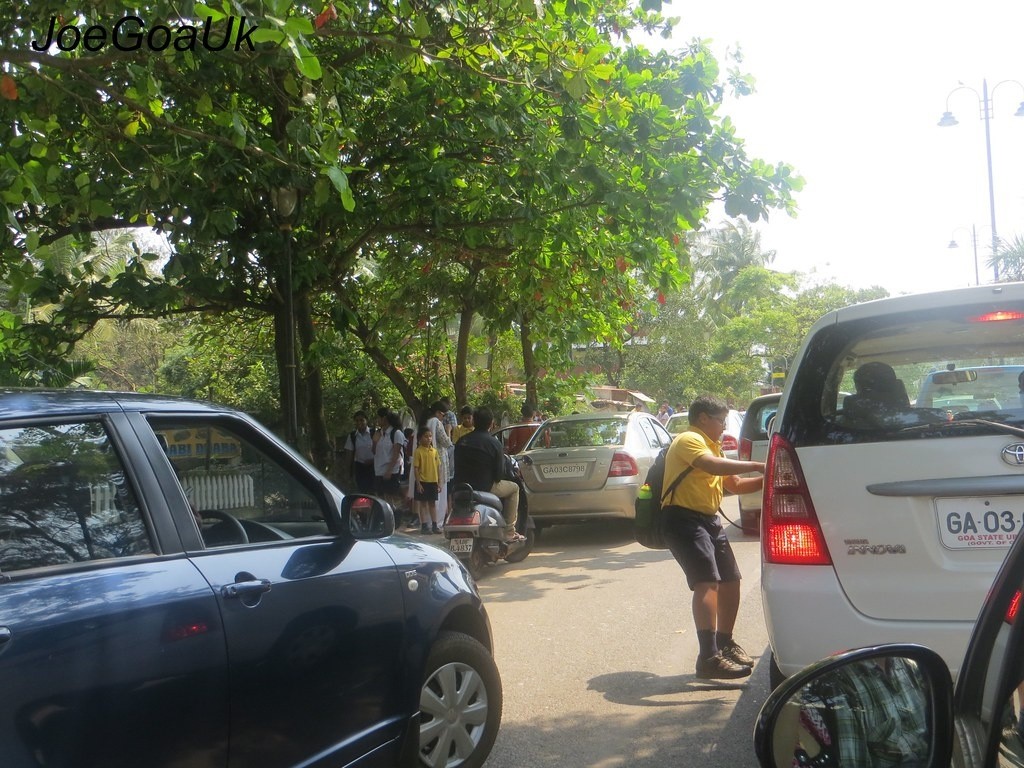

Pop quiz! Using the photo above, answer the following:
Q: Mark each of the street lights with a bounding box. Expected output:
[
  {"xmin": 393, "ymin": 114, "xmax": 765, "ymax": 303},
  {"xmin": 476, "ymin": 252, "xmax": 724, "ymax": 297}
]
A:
[
  {"xmin": 937, "ymin": 80, "xmax": 1024, "ymax": 282},
  {"xmin": 947, "ymin": 223, "xmax": 1000, "ymax": 286}
]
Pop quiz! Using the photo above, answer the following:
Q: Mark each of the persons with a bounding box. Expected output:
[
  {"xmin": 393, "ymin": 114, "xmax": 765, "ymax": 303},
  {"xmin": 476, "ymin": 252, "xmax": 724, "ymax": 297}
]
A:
[
  {"xmin": 345, "ymin": 395, "xmax": 688, "ymax": 545},
  {"xmin": 656, "ymin": 394, "xmax": 767, "ymax": 680},
  {"xmin": 852, "ymin": 361, "xmax": 897, "ymax": 394}
]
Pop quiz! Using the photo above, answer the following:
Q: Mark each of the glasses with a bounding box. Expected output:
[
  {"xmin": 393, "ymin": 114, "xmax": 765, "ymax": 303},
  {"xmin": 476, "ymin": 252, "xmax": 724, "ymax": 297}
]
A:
[
  {"xmin": 440, "ymin": 411, "xmax": 446, "ymax": 417},
  {"xmin": 709, "ymin": 414, "xmax": 725, "ymax": 424}
]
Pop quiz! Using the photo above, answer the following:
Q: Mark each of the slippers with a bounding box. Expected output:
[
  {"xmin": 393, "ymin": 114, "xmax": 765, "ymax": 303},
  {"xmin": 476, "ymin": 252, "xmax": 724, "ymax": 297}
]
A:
[{"xmin": 505, "ymin": 533, "xmax": 528, "ymax": 543}]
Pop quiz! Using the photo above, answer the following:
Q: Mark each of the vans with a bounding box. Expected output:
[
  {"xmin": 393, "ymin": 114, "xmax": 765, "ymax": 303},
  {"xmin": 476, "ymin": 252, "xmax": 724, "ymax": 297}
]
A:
[{"xmin": 760, "ymin": 281, "xmax": 1024, "ymax": 692}]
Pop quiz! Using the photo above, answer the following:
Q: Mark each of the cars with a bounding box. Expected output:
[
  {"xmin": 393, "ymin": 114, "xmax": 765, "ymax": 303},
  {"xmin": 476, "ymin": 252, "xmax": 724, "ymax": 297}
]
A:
[
  {"xmin": 754, "ymin": 516, "xmax": 1024, "ymax": 767},
  {"xmin": 487, "ymin": 409, "xmax": 673, "ymax": 539},
  {"xmin": 916, "ymin": 365, "xmax": 1024, "ymax": 423},
  {"xmin": 663, "ymin": 408, "xmax": 742, "ymax": 461},
  {"xmin": 0, "ymin": 386, "xmax": 501, "ymax": 767},
  {"xmin": 910, "ymin": 396, "xmax": 1001, "ymax": 413},
  {"xmin": 737, "ymin": 391, "xmax": 853, "ymax": 535}
]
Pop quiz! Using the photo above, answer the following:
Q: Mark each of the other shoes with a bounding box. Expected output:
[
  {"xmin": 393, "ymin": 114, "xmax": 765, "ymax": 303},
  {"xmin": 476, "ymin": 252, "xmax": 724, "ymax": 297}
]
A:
[
  {"xmin": 393, "ymin": 508, "xmax": 403, "ymax": 529},
  {"xmin": 407, "ymin": 518, "xmax": 420, "ymax": 528},
  {"xmin": 421, "ymin": 527, "xmax": 432, "ymax": 535},
  {"xmin": 431, "ymin": 527, "xmax": 443, "ymax": 534}
]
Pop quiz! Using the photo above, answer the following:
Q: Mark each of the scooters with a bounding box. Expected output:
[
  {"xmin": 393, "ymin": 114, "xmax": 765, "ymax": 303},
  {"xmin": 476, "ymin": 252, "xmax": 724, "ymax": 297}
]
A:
[{"xmin": 443, "ymin": 454, "xmax": 535, "ymax": 580}]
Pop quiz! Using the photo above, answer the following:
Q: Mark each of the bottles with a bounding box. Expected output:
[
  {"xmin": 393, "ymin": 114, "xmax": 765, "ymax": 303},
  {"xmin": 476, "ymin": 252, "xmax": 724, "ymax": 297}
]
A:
[{"xmin": 638, "ymin": 483, "xmax": 652, "ymax": 499}]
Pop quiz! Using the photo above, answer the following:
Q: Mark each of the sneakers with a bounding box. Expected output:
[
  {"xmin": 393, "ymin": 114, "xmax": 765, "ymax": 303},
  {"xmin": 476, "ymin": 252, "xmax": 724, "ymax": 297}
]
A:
[
  {"xmin": 716, "ymin": 639, "xmax": 755, "ymax": 668},
  {"xmin": 695, "ymin": 649, "xmax": 751, "ymax": 679}
]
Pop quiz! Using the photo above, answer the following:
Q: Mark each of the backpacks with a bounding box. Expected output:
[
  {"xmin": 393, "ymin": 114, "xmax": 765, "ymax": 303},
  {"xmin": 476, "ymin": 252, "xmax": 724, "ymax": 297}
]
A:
[
  {"xmin": 634, "ymin": 443, "xmax": 695, "ymax": 549},
  {"xmin": 390, "ymin": 427, "xmax": 407, "ymax": 482}
]
